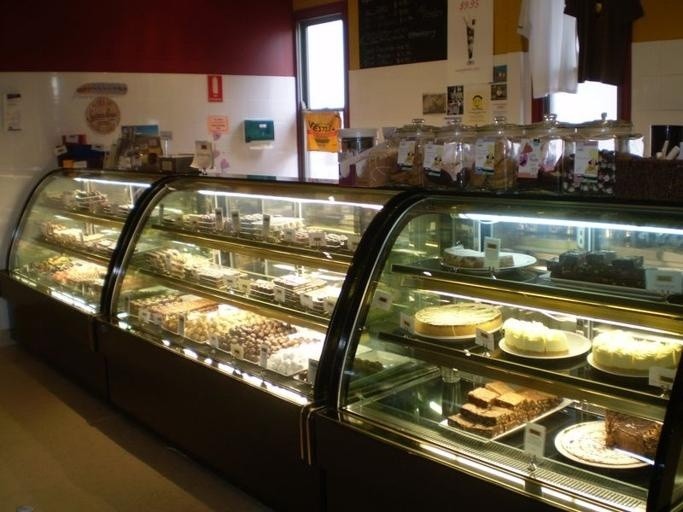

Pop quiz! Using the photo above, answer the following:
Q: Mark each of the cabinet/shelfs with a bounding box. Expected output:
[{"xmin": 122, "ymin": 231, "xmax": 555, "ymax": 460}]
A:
[
  {"xmin": 302, "ymin": 193, "xmax": 683, "ymax": 511},
  {"xmin": 6, "ymin": 169, "xmax": 185, "ymax": 405},
  {"xmin": 93, "ymin": 174, "xmax": 420, "ymax": 511}
]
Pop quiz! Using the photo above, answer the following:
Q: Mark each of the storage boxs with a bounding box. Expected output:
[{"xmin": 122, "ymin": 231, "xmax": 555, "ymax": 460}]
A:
[{"xmin": 615, "ymin": 158, "xmax": 682, "ymax": 205}]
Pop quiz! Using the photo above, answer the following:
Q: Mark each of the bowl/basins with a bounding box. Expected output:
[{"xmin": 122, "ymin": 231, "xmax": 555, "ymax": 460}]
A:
[{"xmin": 157, "ymin": 155, "xmax": 193, "ymax": 173}]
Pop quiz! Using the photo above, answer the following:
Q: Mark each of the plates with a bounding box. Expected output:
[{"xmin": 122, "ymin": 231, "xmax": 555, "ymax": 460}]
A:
[
  {"xmin": 553, "ymin": 419, "xmax": 654, "ymax": 469},
  {"xmin": 439, "ymin": 250, "xmax": 537, "ymax": 275},
  {"xmin": 414, "ymin": 316, "xmax": 505, "ymax": 344},
  {"xmin": 438, "ymin": 396, "xmax": 575, "ymax": 445},
  {"xmin": 585, "ymin": 350, "xmax": 677, "ymax": 379},
  {"xmin": 497, "ymin": 327, "xmax": 593, "ymax": 361}
]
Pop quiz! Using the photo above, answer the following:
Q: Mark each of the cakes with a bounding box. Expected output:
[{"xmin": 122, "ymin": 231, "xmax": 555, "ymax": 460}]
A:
[
  {"xmin": 441, "ymin": 241, "xmax": 514, "ymax": 267},
  {"xmin": 547, "ymin": 249, "xmax": 647, "ymax": 290},
  {"xmin": 448, "ymin": 379, "xmax": 565, "ymax": 440},
  {"xmin": 587, "ymin": 328, "xmax": 682, "ymax": 375},
  {"xmin": 600, "ymin": 406, "xmax": 661, "ymax": 459},
  {"xmin": 500, "ymin": 313, "xmax": 570, "ymax": 356},
  {"xmin": 411, "ymin": 303, "xmax": 504, "ymax": 336}
]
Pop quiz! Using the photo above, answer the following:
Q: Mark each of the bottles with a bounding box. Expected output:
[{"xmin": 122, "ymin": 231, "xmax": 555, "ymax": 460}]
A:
[{"xmin": 335, "ymin": 114, "xmax": 644, "ymax": 201}]
